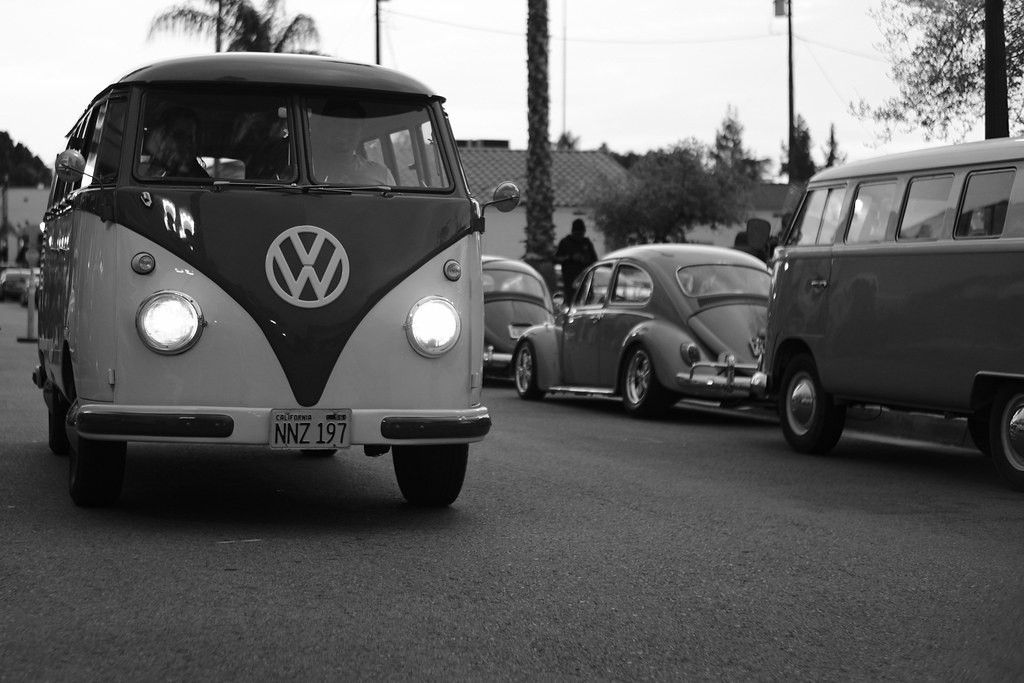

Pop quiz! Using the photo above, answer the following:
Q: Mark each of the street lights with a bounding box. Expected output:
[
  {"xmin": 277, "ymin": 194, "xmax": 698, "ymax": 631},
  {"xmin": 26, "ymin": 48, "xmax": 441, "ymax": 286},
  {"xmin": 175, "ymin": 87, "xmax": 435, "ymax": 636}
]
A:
[{"xmin": 774, "ymin": 0, "xmax": 793, "ymax": 182}]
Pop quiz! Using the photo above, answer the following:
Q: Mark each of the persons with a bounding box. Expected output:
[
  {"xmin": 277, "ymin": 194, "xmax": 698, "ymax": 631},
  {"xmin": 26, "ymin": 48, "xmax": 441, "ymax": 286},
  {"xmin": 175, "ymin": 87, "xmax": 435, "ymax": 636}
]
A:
[
  {"xmin": 551, "ymin": 219, "xmax": 599, "ymax": 309},
  {"xmin": 137, "ymin": 103, "xmax": 267, "ymax": 180},
  {"xmin": 314, "ymin": 106, "xmax": 396, "ymax": 187}
]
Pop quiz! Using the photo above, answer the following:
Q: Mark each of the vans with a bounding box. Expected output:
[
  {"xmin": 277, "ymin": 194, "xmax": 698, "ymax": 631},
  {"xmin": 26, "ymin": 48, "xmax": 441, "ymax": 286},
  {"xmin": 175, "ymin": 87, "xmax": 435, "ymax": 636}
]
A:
[
  {"xmin": 31, "ymin": 53, "xmax": 520, "ymax": 508},
  {"xmin": 768, "ymin": 137, "xmax": 1024, "ymax": 492}
]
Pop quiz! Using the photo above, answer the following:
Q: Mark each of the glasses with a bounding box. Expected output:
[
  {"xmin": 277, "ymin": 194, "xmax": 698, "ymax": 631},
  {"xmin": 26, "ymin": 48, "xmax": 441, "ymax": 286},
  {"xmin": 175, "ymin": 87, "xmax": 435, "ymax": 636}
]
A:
[{"xmin": 169, "ymin": 130, "xmax": 200, "ymax": 142}]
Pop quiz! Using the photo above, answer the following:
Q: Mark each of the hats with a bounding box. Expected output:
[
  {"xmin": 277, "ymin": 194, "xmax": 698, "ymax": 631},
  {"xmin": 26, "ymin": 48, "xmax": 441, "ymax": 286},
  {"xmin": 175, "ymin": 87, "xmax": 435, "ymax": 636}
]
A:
[{"xmin": 572, "ymin": 219, "xmax": 585, "ymax": 232}]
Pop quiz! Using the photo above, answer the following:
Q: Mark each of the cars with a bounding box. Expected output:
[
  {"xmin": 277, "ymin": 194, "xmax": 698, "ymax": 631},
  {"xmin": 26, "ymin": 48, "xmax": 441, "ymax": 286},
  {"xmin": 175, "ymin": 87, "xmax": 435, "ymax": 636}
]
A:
[
  {"xmin": 512, "ymin": 244, "xmax": 774, "ymax": 416},
  {"xmin": 481, "ymin": 255, "xmax": 556, "ymax": 369},
  {"xmin": 0, "ymin": 268, "xmax": 39, "ymax": 305}
]
